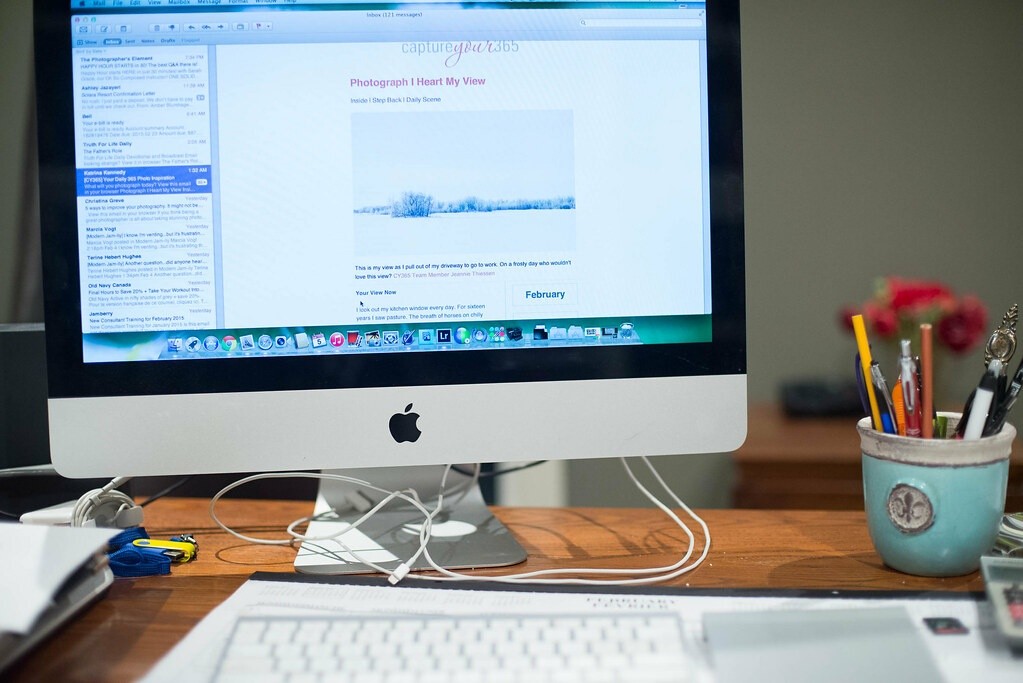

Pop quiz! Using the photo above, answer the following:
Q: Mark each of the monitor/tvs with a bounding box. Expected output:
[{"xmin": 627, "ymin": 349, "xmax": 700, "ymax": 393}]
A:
[{"xmin": 34, "ymin": 1, "xmax": 750, "ymax": 576}]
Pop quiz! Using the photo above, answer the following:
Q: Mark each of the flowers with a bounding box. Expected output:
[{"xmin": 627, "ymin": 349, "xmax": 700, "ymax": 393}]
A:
[{"xmin": 842, "ymin": 279, "xmax": 988, "ymax": 355}]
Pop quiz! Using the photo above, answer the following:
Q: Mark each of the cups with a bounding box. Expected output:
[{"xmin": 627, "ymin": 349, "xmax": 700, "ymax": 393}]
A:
[{"xmin": 857, "ymin": 412, "xmax": 1017, "ymax": 579}]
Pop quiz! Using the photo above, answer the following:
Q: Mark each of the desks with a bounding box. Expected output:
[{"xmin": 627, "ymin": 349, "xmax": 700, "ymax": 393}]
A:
[
  {"xmin": 732, "ymin": 402, "xmax": 1023, "ymax": 508},
  {"xmin": 0, "ymin": 496, "xmax": 1023, "ymax": 683}
]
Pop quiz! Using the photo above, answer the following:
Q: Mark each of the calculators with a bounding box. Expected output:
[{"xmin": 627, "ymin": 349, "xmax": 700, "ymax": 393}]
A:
[{"xmin": 980, "ymin": 554, "xmax": 1023, "ymax": 654}]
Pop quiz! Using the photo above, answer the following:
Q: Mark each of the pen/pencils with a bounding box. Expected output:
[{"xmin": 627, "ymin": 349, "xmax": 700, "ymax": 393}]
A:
[
  {"xmin": 850, "ymin": 312, "xmax": 936, "ymax": 438},
  {"xmin": 956, "ymin": 356, "xmax": 1023, "ymax": 439}
]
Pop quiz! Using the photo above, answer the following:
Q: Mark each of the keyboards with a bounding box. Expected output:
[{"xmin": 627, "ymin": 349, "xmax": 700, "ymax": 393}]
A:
[{"xmin": 205, "ymin": 609, "xmax": 700, "ymax": 683}]
